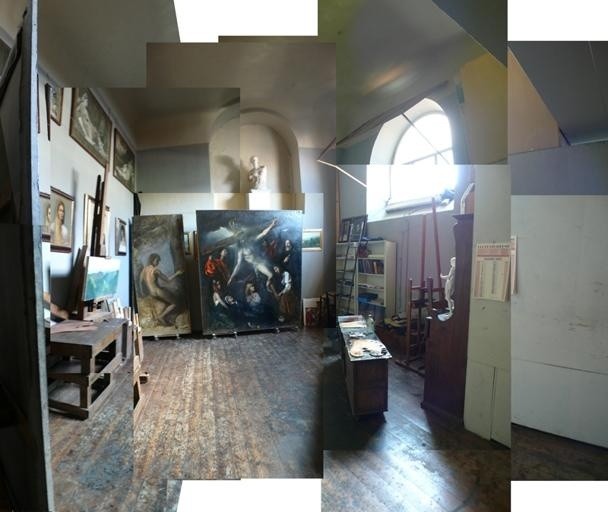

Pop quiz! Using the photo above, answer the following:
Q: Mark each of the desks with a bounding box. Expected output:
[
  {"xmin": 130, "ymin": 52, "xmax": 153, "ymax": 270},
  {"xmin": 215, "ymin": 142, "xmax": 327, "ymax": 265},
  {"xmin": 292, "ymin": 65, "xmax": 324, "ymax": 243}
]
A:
[
  {"xmin": 50, "ymin": 315, "xmax": 133, "ymax": 422},
  {"xmin": 336, "ymin": 314, "xmax": 393, "ymax": 417}
]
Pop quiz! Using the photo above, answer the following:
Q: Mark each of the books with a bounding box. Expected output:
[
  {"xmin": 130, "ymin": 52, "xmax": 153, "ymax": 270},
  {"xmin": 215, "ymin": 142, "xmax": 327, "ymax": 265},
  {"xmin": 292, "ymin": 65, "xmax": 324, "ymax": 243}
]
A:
[{"xmin": 358, "ymin": 259, "xmax": 385, "ymax": 274}]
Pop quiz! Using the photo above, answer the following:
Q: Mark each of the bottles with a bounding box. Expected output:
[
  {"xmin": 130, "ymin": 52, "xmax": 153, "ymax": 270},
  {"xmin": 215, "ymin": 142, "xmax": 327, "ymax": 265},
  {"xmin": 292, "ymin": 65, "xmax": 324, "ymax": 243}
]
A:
[{"xmin": 367, "ymin": 314, "xmax": 374, "ymax": 332}]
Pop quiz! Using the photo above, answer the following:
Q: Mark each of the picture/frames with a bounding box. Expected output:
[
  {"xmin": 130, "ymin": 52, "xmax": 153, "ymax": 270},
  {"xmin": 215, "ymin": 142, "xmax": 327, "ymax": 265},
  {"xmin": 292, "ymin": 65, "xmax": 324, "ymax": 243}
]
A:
[
  {"xmin": 113, "ymin": 129, "xmax": 136, "ymax": 192},
  {"xmin": 69, "ymin": 87, "xmax": 111, "ymax": 168},
  {"xmin": 51, "ymin": 186, "xmax": 77, "ymax": 253},
  {"xmin": 50, "ymin": 88, "xmax": 65, "ymax": 127},
  {"xmin": 114, "ymin": 218, "xmax": 128, "ymax": 256},
  {"xmin": 84, "ymin": 193, "xmax": 109, "ymax": 258},
  {"xmin": 39, "ymin": 186, "xmax": 50, "ymax": 242}
]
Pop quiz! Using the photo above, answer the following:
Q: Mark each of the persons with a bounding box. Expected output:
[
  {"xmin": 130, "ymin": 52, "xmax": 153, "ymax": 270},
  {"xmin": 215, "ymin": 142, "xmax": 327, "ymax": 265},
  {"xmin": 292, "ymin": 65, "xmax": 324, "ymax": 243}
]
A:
[
  {"xmin": 139, "ymin": 253, "xmax": 184, "ymax": 327},
  {"xmin": 44, "ymin": 204, "xmax": 51, "ymax": 235},
  {"xmin": 439, "ymin": 257, "xmax": 456, "ymax": 316},
  {"xmin": 203, "ymin": 217, "xmax": 299, "ymax": 323},
  {"xmin": 51, "ymin": 200, "xmax": 68, "ymax": 245}
]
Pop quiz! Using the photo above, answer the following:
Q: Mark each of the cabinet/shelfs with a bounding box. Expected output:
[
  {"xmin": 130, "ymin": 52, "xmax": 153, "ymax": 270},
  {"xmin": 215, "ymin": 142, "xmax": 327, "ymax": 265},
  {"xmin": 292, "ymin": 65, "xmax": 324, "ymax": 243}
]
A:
[
  {"xmin": 132, "ymin": 316, "xmax": 145, "ymax": 420},
  {"xmin": 335, "ymin": 240, "xmax": 397, "ymax": 323}
]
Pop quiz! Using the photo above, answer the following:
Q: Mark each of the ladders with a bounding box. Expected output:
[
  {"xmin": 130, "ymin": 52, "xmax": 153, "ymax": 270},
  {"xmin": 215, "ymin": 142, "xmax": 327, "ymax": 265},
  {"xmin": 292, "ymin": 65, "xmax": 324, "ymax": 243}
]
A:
[{"xmin": 338, "ymin": 218, "xmax": 364, "ymax": 316}]
